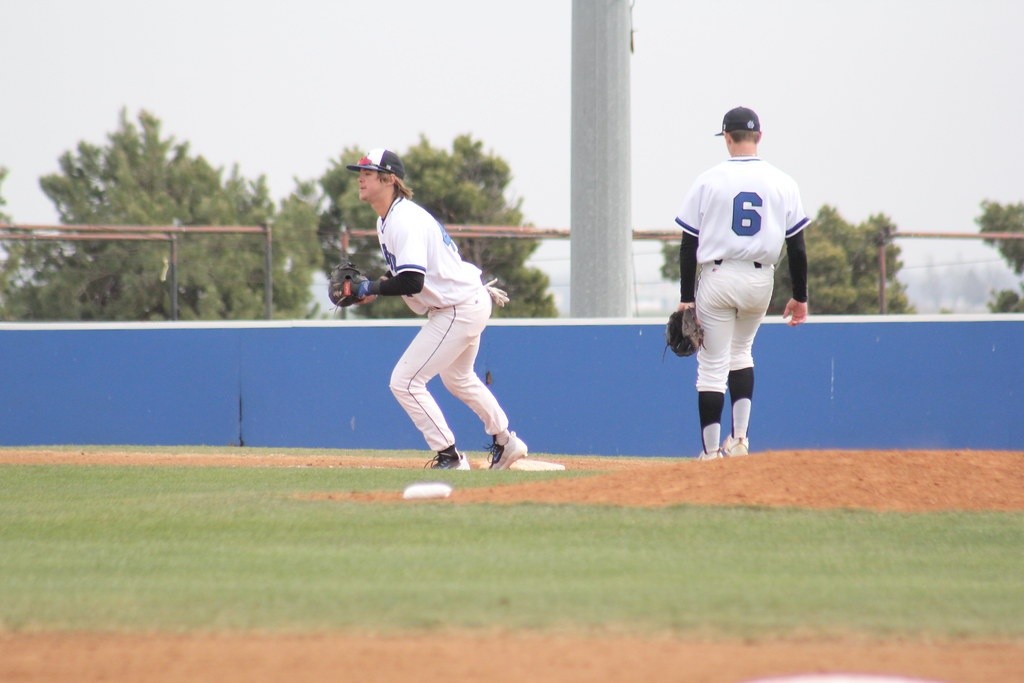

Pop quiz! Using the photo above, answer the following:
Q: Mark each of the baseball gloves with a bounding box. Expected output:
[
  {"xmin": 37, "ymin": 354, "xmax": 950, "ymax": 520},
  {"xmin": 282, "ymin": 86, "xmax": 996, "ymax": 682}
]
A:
[
  {"xmin": 666, "ymin": 307, "xmax": 705, "ymax": 358},
  {"xmin": 327, "ymin": 260, "xmax": 368, "ymax": 308}
]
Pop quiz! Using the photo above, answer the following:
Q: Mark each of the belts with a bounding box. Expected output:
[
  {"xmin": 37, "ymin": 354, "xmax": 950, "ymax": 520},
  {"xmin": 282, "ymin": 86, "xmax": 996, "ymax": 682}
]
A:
[
  {"xmin": 715, "ymin": 259, "xmax": 761, "ymax": 268},
  {"xmin": 434, "ymin": 307, "xmax": 439, "ymax": 310}
]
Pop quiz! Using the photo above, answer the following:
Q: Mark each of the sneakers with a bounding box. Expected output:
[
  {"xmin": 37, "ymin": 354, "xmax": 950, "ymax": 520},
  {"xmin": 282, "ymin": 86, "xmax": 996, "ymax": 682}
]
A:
[
  {"xmin": 483, "ymin": 431, "xmax": 528, "ymax": 470},
  {"xmin": 423, "ymin": 453, "xmax": 470, "ymax": 471},
  {"xmin": 699, "ymin": 448, "xmax": 723, "ymax": 460},
  {"xmin": 722, "ymin": 434, "xmax": 749, "ymax": 457}
]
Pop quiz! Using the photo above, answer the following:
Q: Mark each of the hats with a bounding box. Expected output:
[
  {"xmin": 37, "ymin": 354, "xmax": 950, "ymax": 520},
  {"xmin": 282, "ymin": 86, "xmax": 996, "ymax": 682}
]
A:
[
  {"xmin": 715, "ymin": 106, "xmax": 760, "ymax": 136},
  {"xmin": 346, "ymin": 149, "xmax": 405, "ymax": 180}
]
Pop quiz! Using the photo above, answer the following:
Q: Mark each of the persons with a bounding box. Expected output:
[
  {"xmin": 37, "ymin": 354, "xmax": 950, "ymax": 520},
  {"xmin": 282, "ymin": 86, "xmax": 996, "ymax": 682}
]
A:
[
  {"xmin": 328, "ymin": 148, "xmax": 529, "ymax": 470},
  {"xmin": 674, "ymin": 107, "xmax": 812, "ymax": 461}
]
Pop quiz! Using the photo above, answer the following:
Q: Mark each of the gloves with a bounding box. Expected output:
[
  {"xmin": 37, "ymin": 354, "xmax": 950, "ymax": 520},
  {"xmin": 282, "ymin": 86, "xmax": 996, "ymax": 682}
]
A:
[{"xmin": 484, "ymin": 277, "xmax": 510, "ymax": 308}]
